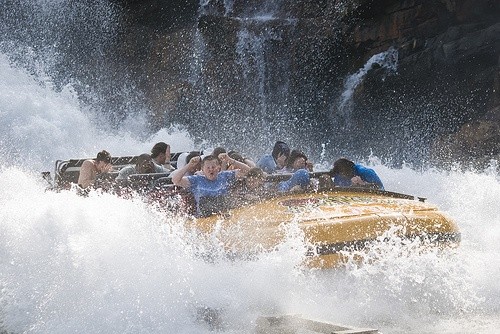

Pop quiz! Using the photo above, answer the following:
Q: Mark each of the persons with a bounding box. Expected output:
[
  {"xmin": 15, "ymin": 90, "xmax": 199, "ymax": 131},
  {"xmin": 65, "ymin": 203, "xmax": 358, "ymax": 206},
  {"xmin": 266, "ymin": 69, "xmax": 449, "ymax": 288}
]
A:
[
  {"xmin": 77, "ymin": 149, "xmax": 114, "ymax": 192},
  {"xmin": 117, "ymin": 153, "xmax": 172, "ymax": 191},
  {"xmin": 146, "ymin": 142, "xmax": 177, "ymax": 176},
  {"xmin": 170, "ymin": 142, "xmax": 385, "ymax": 225}
]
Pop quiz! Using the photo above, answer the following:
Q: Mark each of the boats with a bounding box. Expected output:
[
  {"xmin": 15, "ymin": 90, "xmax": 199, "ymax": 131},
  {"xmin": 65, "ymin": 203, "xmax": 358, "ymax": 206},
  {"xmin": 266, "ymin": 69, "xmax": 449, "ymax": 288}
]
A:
[{"xmin": 42, "ymin": 153, "xmax": 462, "ymax": 272}]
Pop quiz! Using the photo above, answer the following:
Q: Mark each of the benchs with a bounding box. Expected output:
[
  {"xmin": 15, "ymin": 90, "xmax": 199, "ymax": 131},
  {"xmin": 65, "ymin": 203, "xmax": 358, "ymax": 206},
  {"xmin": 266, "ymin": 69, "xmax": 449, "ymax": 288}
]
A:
[{"xmin": 58, "ymin": 151, "xmax": 330, "ymax": 216}]
